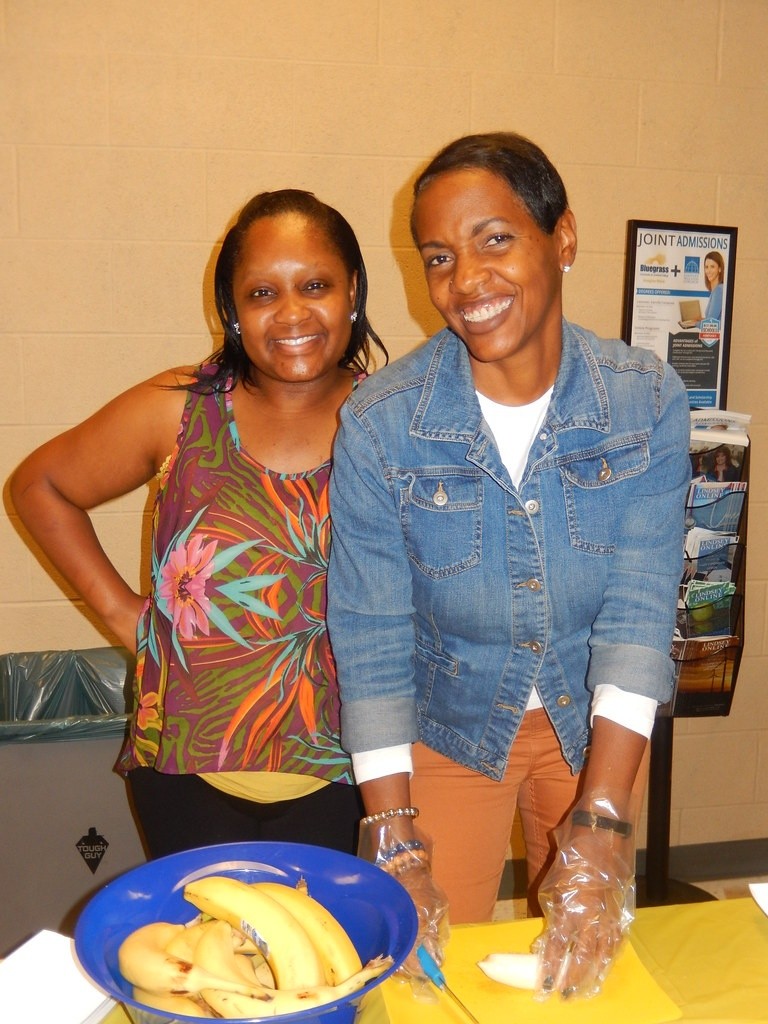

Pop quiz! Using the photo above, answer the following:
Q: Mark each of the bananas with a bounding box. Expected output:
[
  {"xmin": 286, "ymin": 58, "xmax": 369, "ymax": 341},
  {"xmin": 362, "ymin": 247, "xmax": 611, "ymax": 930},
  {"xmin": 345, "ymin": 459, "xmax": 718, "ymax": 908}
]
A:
[
  {"xmin": 117, "ymin": 874, "xmax": 395, "ymax": 1020},
  {"xmin": 479, "ymin": 951, "xmax": 574, "ymax": 989}
]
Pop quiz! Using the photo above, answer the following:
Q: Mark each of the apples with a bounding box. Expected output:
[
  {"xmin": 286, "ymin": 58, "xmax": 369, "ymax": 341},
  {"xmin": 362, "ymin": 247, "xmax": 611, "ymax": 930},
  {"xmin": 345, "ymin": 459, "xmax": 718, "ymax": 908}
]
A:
[{"xmin": 691, "ymin": 602, "xmax": 714, "ymax": 634}]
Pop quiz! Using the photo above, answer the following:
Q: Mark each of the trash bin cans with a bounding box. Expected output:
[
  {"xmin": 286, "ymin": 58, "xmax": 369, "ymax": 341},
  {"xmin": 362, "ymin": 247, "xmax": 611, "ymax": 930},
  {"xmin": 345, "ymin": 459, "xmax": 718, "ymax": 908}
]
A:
[{"xmin": 0, "ymin": 645, "xmax": 147, "ymax": 960}]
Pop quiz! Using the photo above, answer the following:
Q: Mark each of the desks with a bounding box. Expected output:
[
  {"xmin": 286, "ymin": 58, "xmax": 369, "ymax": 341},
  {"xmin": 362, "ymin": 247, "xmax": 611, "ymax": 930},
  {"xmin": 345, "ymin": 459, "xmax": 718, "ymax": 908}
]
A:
[{"xmin": 89, "ymin": 899, "xmax": 768, "ymax": 1024}]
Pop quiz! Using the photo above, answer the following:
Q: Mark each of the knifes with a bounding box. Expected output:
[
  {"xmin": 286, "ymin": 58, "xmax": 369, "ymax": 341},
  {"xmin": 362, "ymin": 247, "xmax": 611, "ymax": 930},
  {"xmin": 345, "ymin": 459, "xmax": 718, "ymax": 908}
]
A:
[{"xmin": 417, "ymin": 944, "xmax": 481, "ymax": 1024}]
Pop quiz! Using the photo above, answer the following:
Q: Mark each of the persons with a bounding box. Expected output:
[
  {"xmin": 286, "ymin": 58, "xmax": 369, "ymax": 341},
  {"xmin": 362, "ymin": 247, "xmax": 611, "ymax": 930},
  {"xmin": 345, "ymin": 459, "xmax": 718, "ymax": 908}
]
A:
[
  {"xmin": 326, "ymin": 130, "xmax": 693, "ymax": 999},
  {"xmin": 10, "ymin": 189, "xmax": 389, "ymax": 860},
  {"xmin": 703, "ymin": 251, "xmax": 724, "ymax": 322},
  {"xmin": 695, "ymin": 444, "xmax": 741, "ymax": 482}
]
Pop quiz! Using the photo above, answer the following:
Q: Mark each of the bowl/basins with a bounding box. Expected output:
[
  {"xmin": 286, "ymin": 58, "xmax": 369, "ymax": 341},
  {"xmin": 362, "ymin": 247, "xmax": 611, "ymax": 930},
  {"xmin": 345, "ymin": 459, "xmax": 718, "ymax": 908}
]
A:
[{"xmin": 74, "ymin": 841, "xmax": 419, "ymax": 1024}]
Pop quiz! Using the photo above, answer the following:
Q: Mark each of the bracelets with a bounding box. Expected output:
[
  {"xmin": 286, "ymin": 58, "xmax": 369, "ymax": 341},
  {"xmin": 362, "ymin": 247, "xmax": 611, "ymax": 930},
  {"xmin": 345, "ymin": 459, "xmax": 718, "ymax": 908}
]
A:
[
  {"xmin": 359, "ymin": 806, "xmax": 420, "ymax": 824},
  {"xmin": 373, "ymin": 840, "xmax": 432, "ymax": 877},
  {"xmin": 572, "ymin": 809, "xmax": 632, "ymax": 839}
]
